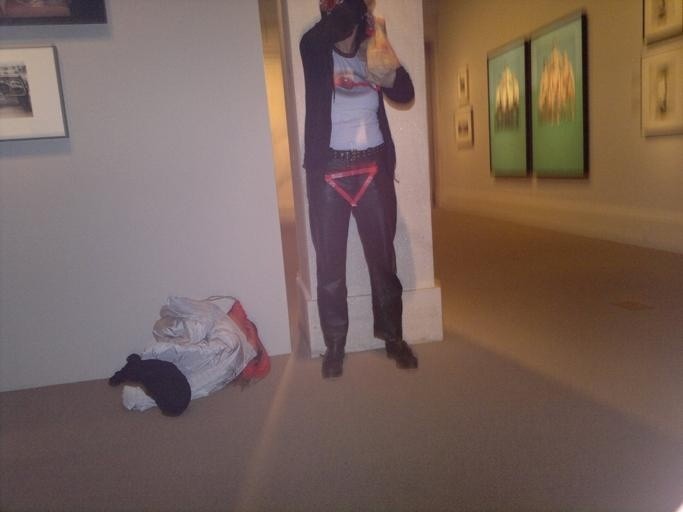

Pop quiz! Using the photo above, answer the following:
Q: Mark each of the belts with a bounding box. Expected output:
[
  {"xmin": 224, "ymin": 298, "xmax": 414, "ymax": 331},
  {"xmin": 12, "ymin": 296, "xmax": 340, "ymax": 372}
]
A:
[{"xmin": 331, "ymin": 144, "xmax": 388, "ymax": 160}]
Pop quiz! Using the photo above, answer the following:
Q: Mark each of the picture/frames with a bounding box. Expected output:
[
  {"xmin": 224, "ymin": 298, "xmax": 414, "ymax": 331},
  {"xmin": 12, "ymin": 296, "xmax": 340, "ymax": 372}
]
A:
[
  {"xmin": 643, "ymin": 1, "xmax": 681, "ymax": 45},
  {"xmin": 529, "ymin": 9, "xmax": 590, "ymax": 179},
  {"xmin": 456, "ymin": 64, "xmax": 473, "ymax": 149},
  {"xmin": 486, "ymin": 34, "xmax": 532, "ymax": 177},
  {"xmin": 640, "ymin": 36, "xmax": 681, "ymax": 137},
  {"xmin": 0, "ymin": 0, "xmax": 108, "ymax": 140}
]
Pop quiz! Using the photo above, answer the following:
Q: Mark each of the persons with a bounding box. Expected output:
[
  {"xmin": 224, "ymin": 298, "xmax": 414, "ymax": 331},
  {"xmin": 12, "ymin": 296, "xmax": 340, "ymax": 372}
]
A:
[
  {"xmin": 537, "ymin": 43, "xmax": 575, "ymax": 123},
  {"xmin": 300, "ymin": 1, "xmax": 418, "ymax": 378}
]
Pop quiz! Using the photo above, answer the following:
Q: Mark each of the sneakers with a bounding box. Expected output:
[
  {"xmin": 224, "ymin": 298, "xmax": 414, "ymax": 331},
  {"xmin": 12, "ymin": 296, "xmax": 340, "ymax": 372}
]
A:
[
  {"xmin": 388, "ymin": 339, "xmax": 417, "ymax": 369},
  {"xmin": 322, "ymin": 348, "xmax": 344, "ymax": 377}
]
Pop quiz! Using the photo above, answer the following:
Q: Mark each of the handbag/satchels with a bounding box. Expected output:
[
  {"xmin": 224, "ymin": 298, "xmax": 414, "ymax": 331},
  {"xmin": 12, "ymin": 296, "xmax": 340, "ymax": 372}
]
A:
[{"xmin": 208, "ymin": 296, "xmax": 270, "ymax": 384}]
